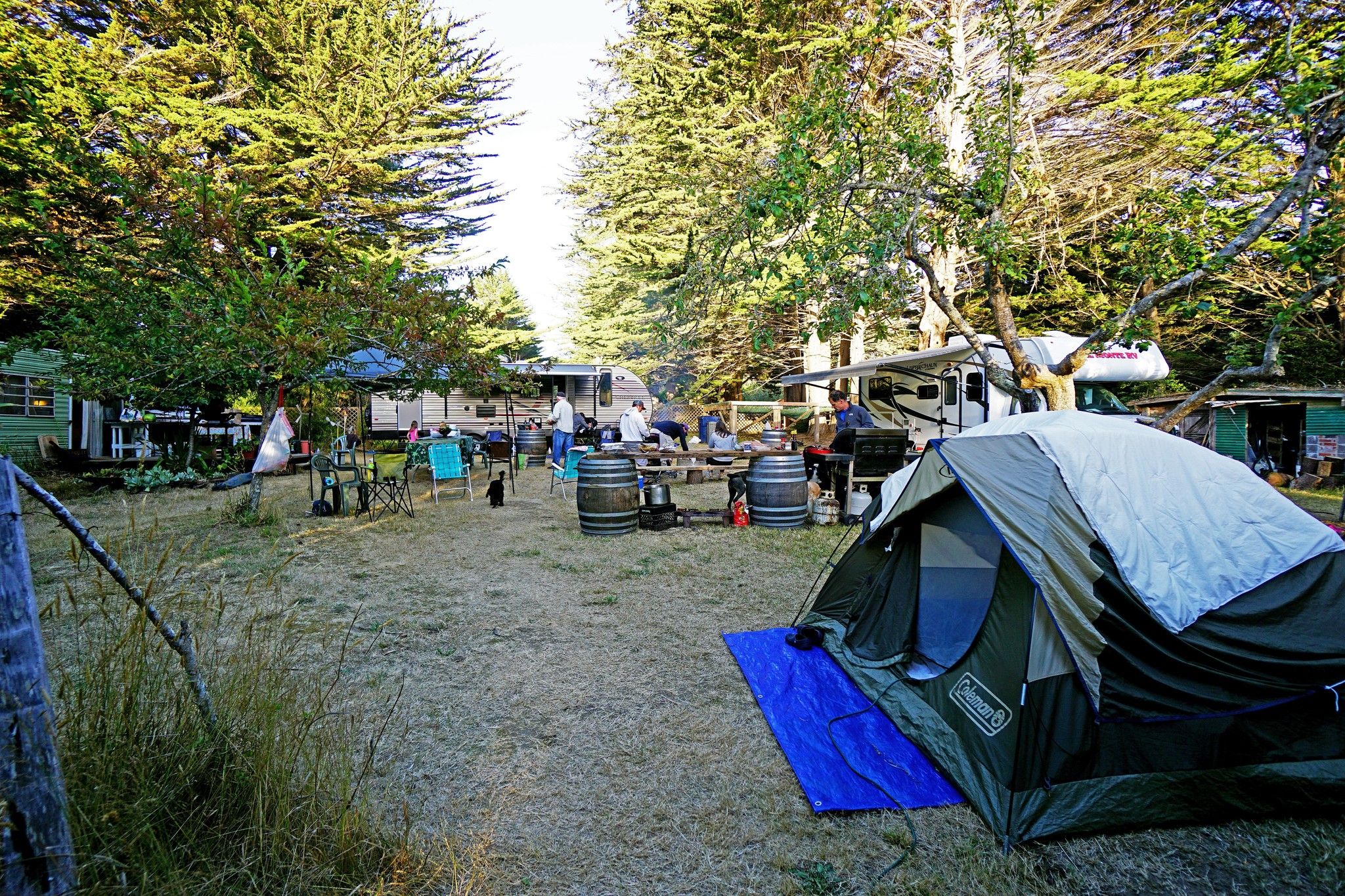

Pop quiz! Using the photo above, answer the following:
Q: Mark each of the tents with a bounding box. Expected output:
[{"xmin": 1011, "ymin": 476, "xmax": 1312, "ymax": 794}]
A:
[
  {"xmin": 307, "ymin": 341, "xmax": 519, "ymax": 503},
  {"xmin": 720, "ymin": 410, "xmax": 1345, "ymax": 859}
]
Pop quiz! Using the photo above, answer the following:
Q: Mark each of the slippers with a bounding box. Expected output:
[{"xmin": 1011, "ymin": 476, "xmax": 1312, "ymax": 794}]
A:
[{"xmin": 644, "ymin": 480, "xmax": 653, "ymax": 485}]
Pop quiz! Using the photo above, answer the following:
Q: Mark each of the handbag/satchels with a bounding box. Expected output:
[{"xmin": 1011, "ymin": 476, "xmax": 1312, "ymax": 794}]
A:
[
  {"xmin": 251, "ymin": 385, "xmax": 296, "ymax": 473},
  {"xmin": 312, "ymin": 499, "xmax": 332, "ymax": 516}
]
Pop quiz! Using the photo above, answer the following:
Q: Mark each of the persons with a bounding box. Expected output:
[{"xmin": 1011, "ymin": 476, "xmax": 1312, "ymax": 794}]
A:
[
  {"xmin": 650, "ymin": 420, "xmax": 692, "ymax": 476},
  {"xmin": 828, "ymin": 390, "xmax": 874, "ymax": 434},
  {"xmin": 547, "ymin": 392, "xmax": 573, "ymax": 470},
  {"xmin": 619, "ymin": 400, "xmax": 654, "ymax": 484},
  {"xmin": 407, "ymin": 420, "xmax": 418, "ymax": 442},
  {"xmin": 572, "ymin": 413, "xmax": 591, "ymax": 438},
  {"xmin": 705, "ymin": 421, "xmax": 734, "ymax": 481},
  {"xmin": 439, "ymin": 422, "xmax": 446, "ymax": 428}
]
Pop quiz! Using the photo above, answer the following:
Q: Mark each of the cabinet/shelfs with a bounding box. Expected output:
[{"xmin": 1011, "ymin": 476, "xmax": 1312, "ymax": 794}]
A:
[
  {"xmin": 1299, "ymin": 417, "xmax": 1306, "ymax": 465},
  {"xmin": 1266, "ymin": 418, "xmax": 1282, "ymax": 464}
]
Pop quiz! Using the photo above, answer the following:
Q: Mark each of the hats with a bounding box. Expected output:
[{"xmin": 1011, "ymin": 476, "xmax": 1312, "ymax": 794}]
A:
[
  {"xmin": 555, "ymin": 392, "xmax": 565, "ymax": 397},
  {"xmin": 680, "ymin": 423, "xmax": 688, "ymax": 436},
  {"xmin": 633, "ymin": 400, "xmax": 646, "ymax": 410}
]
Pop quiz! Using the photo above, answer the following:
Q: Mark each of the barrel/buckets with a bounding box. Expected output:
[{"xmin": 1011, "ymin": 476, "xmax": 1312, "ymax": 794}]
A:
[
  {"xmin": 744, "ymin": 443, "xmax": 751, "ymax": 452},
  {"xmin": 577, "ymin": 457, "xmax": 640, "ymax": 537},
  {"xmin": 300, "ymin": 441, "xmax": 313, "ymax": 454},
  {"xmin": 517, "ymin": 430, "xmax": 547, "ymax": 467},
  {"xmin": 761, "ymin": 430, "xmax": 787, "ymax": 448},
  {"xmin": 1247, "ymin": 462, "xmax": 1275, "ymax": 474},
  {"xmin": 1296, "ymin": 465, "xmax": 1301, "ymax": 478},
  {"xmin": 746, "ymin": 454, "xmax": 808, "ymax": 531}
]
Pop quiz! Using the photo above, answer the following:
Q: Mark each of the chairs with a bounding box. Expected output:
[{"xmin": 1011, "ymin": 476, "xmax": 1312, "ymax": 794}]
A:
[
  {"xmin": 592, "ymin": 430, "xmax": 615, "ymax": 451},
  {"xmin": 486, "ymin": 430, "xmax": 502, "ymax": 456},
  {"xmin": 355, "ymin": 453, "xmax": 415, "ymax": 524},
  {"xmin": 467, "ymin": 434, "xmax": 491, "ymax": 471},
  {"xmin": 549, "ymin": 446, "xmax": 595, "ymax": 503},
  {"xmin": 487, "ymin": 441, "xmax": 518, "ymax": 480},
  {"xmin": 311, "ymin": 455, "xmax": 368, "ymax": 518},
  {"xmin": 428, "ymin": 444, "xmax": 474, "ymax": 504},
  {"xmin": 331, "ymin": 434, "xmax": 361, "ymax": 467}
]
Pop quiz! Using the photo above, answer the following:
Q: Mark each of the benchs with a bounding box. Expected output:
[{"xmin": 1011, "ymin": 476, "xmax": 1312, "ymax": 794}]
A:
[{"xmin": 635, "ymin": 464, "xmax": 749, "ymax": 485}]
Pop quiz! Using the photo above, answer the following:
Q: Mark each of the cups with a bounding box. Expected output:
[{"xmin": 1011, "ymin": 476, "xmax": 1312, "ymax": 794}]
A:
[{"xmin": 775, "ymin": 427, "xmax": 778, "ymax": 429}]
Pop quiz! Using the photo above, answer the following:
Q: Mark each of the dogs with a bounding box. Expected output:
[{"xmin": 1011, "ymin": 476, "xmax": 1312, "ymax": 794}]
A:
[
  {"xmin": 803, "ymin": 445, "xmax": 831, "ymax": 490},
  {"xmin": 805, "ymin": 481, "xmax": 820, "ymax": 517},
  {"xmin": 486, "ymin": 470, "xmax": 506, "ymax": 508},
  {"xmin": 727, "ymin": 470, "xmax": 747, "ymax": 511}
]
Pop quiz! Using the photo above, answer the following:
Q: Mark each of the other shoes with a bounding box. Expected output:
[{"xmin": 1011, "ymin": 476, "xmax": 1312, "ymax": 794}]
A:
[
  {"xmin": 708, "ymin": 475, "xmax": 713, "ymax": 480},
  {"xmin": 714, "ymin": 474, "xmax": 723, "ymax": 480},
  {"xmin": 664, "ymin": 470, "xmax": 679, "ymax": 475},
  {"xmin": 547, "ymin": 466, "xmax": 556, "ymax": 469}
]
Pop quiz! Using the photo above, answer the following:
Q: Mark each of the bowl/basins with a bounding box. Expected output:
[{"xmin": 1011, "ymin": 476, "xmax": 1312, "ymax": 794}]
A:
[{"xmin": 636, "ymin": 443, "xmax": 658, "ymax": 453}]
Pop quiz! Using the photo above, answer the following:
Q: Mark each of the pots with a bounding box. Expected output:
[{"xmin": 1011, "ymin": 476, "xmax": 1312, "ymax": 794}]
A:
[{"xmin": 642, "ymin": 484, "xmax": 671, "ymax": 506}]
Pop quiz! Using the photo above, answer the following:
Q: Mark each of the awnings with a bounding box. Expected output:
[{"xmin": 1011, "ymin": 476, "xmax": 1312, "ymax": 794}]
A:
[
  {"xmin": 445, "ymin": 363, "xmax": 606, "ymax": 420},
  {"xmin": 782, "ymin": 343, "xmax": 987, "ymax": 391}
]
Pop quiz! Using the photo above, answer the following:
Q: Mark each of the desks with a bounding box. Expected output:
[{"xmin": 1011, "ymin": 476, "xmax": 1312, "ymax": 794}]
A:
[
  {"xmin": 194, "ymin": 423, "xmax": 246, "ymax": 459},
  {"xmin": 104, "ymin": 422, "xmax": 160, "ymax": 458},
  {"xmin": 405, "ymin": 436, "xmax": 474, "ymax": 483}
]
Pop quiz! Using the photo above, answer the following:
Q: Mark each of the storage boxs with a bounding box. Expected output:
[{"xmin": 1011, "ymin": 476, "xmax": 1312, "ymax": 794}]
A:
[
  {"xmin": 638, "ymin": 503, "xmax": 679, "ymax": 532},
  {"xmin": 200, "ymin": 446, "xmax": 217, "ymax": 459}
]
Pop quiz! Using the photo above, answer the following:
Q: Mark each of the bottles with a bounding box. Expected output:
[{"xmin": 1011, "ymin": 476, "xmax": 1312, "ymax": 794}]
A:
[
  {"xmin": 548, "ymin": 447, "xmax": 552, "ymax": 454},
  {"xmin": 731, "ymin": 433, "xmax": 737, "ymax": 450},
  {"xmin": 785, "ymin": 442, "xmax": 792, "ymax": 450},
  {"xmin": 792, "ymin": 434, "xmax": 797, "ymax": 451},
  {"xmin": 532, "ymin": 418, "xmax": 534, "ymax": 424},
  {"xmin": 291, "ymin": 440, "xmax": 298, "ymax": 454},
  {"xmin": 785, "ymin": 439, "xmax": 788, "ymax": 442},
  {"xmin": 789, "ymin": 437, "xmax": 792, "ymax": 442},
  {"xmin": 457, "ymin": 429, "xmax": 461, "ymax": 437},
  {"xmin": 765, "ymin": 421, "xmax": 769, "ymax": 429},
  {"xmin": 523, "ymin": 418, "xmax": 527, "ymax": 424},
  {"xmin": 317, "ymin": 448, "xmax": 320, "ymax": 453},
  {"xmin": 782, "ymin": 425, "xmax": 784, "ymax": 429},
  {"xmin": 781, "ymin": 438, "xmax": 785, "ymax": 449},
  {"xmin": 528, "ymin": 419, "xmax": 530, "ymax": 424}
]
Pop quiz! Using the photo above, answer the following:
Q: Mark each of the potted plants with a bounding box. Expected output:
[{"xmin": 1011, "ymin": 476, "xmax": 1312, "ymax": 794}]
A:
[{"xmin": 236, "ymin": 434, "xmax": 260, "ymax": 459}]
